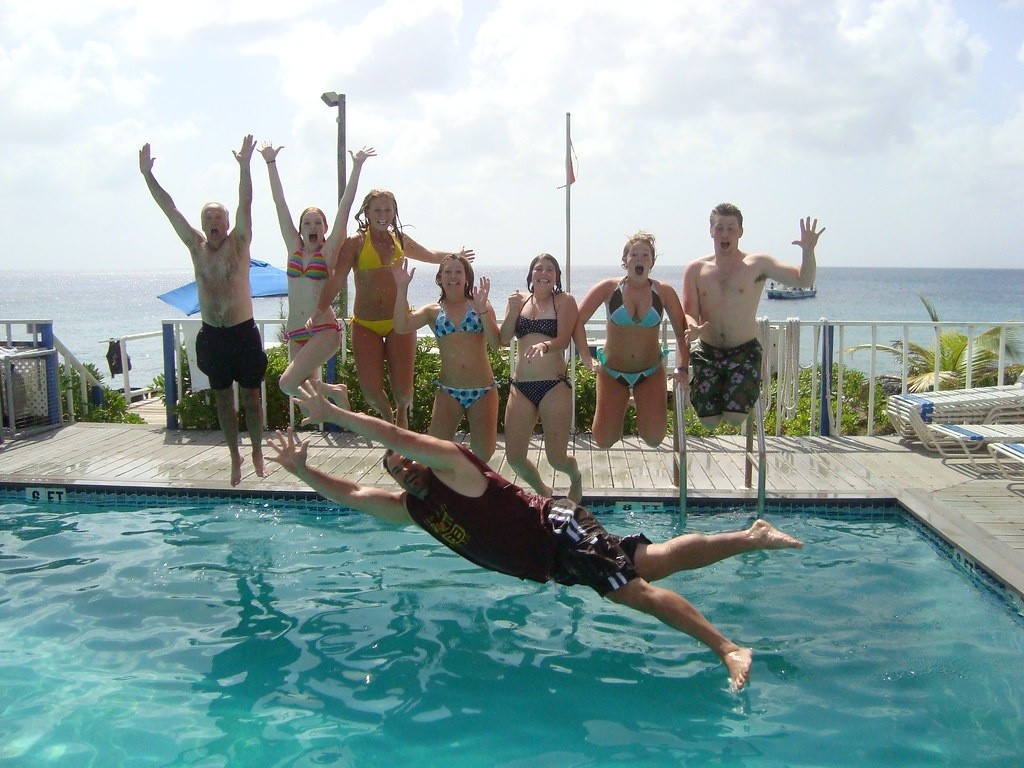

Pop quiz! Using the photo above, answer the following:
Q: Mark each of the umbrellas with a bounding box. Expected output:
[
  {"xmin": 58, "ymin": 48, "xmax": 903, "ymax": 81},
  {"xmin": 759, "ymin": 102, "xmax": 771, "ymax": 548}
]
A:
[{"xmin": 156, "ymin": 257, "xmax": 288, "ymax": 315}]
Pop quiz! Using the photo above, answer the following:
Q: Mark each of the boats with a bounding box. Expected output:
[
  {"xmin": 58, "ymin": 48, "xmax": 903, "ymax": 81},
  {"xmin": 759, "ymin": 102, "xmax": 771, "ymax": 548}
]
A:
[{"xmin": 766, "ymin": 282, "xmax": 817, "ymax": 299}]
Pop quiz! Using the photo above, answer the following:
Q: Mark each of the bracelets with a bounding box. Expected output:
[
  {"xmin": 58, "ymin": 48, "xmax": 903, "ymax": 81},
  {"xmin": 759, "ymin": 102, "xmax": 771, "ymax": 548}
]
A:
[
  {"xmin": 480, "ymin": 311, "xmax": 488, "ymax": 315},
  {"xmin": 543, "ymin": 342, "xmax": 548, "ymax": 353},
  {"xmin": 267, "ymin": 160, "xmax": 276, "ymax": 164}
]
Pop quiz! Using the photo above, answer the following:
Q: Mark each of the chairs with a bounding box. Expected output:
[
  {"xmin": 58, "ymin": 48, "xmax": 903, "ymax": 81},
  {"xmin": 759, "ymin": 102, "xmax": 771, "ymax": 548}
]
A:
[{"xmin": 884, "ymin": 370, "xmax": 1023, "ymax": 482}]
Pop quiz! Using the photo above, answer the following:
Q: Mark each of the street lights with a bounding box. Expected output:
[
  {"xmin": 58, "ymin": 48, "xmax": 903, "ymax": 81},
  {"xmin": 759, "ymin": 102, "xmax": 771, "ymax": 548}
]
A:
[{"xmin": 322, "ymin": 92, "xmax": 348, "ymax": 318}]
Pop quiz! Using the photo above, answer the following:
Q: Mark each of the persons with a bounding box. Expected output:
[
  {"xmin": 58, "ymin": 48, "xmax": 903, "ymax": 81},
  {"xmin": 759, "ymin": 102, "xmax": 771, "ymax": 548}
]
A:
[
  {"xmin": 304, "ymin": 190, "xmax": 475, "ymax": 428},
  {"xmin": 139, "ymin": 134, "xmax": 268, "ymax": 487},
  {"xmin": 256, "ymin": 140, "xmax": 376, "ymax": 412},
  {"xmin": 574, "ymin": 232, "xmax": 689, "ymax": 448},
  {"xmin": 500, "ymin": 254, "xmax": 582, "ymax": 504},
  {"xmin": 263, "ymin": 379, "xmax": 803, "ymax": 693},
  {"xmin": 392, "ymin": 253, "xmax": 499, "ymax": 463},
  {"xmin": 683, "ymin": 203, "xmax": 826, "ymax": 429}
]
